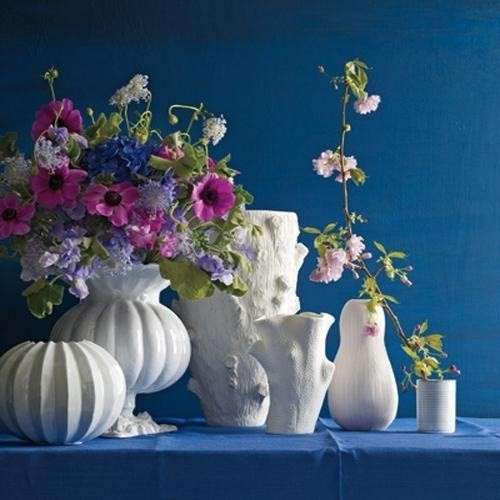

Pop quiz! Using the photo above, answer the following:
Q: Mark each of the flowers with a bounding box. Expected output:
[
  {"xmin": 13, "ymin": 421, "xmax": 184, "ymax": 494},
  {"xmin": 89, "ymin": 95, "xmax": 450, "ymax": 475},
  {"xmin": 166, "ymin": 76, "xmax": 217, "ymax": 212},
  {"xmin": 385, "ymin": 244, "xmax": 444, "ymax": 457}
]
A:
[
  {"xmin": 0, "ymin": 66, "xmax": 269, "ymax": 318},
  {"xmin": 300, "ymin": 57, "xmax": 462, "ymax": 393}
]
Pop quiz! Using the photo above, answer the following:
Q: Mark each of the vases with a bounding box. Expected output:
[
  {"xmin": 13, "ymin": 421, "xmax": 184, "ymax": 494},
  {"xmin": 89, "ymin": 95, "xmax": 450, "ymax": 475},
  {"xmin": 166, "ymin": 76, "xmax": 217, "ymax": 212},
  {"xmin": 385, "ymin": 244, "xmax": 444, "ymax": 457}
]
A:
[
  {"xmin": 0, "ymin": 342, "xmax": 126, "ymax": 446},
  {"xmin": 327, "ymin": 298, "xmax": 400, "ymax": 430},
  {"xmin": 248, "ymin": 312, "xmax": 335, "ymax": 435},
  {"xmin": 48, "ymin": 264, "xmax": 192, "ymax": 437},
  {"xmin": 168, "ymin": 210, "xmax": 310, "ymax": 427},
  {"xmin": 417, "ymin": 380, "xmax": 457, "ymax": 433}
]
